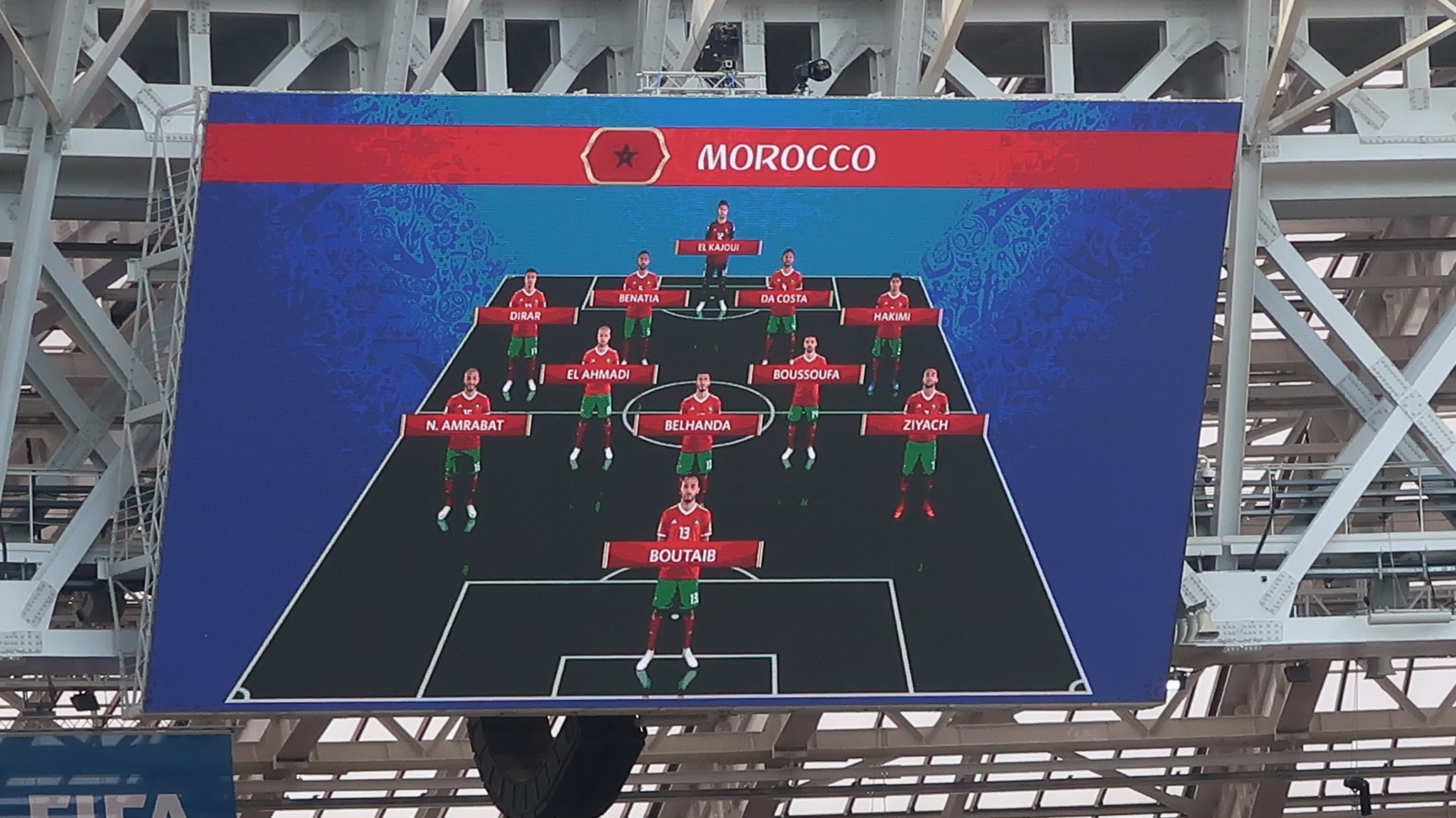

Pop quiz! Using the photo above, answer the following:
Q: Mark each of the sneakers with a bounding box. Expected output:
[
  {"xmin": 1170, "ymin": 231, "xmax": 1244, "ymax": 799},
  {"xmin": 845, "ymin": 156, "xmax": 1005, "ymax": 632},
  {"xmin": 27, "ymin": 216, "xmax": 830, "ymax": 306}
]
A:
[
  {"xmin": 789, "ymin": 359, "xmax": 795, "ymax": 366},
  {"xmin": 718, "ymin": 298, "xmax": 727, "ymax": 312},
  {"xmin": 921, "ymin": 501, "xmax": 935, "ymax": 517},
  {"xmin": 681, "ymin": 648, "xmax": 699, "ymax": 668},
  {"xmin": 570, "ymin": 447, "xmax": 580, "ymax": 461},
  {"xmin": 867, "ymin": 380, "xmax": 877, "ymax": 395},
  {"xmin": 502, "ymin": 380, "xmax": 513, "ymax": 392},
  {"xmin": 893, "ymin": 504, "xmax": 905, "ymax": 519},
  {"xmin": 781, "ymin": 448, "xmax": 794, "ymax": 461},
  {"xmin": 641, "ymin": 358, "xmax": 648, "ymax": 366},
  {"xmin": 605, "ymin": 447, "xmax": 613, "ymax": 459},
  {"xmin": 761, "ymin": 360, "xmax": 768, "ymax": 366},
  {"xmin": 467, "ymin": 504, "xmax": 477, "ymax": 518},
  {"xmin": 636, "ymin": 649, "xmax": 655, "ymax": 671},
  {"xmin": 807, "ymin": 446, "xmax": 816, "ymax": 460},
  {"xmin": 527, "ymin": 379, "xmax": 537, "ymax": 391},
  {"xmin": 621, "ymin": 360, "xmax": 627, "ymax": 366},
  {"xmin": 696, "ymin": 301, "xmax": 706, "ymax": 312},
  {"xmin": 891, "ymin": 379, "xmax": 899, "ymax": 390},
  {"xmin": 438, "ymin": 505, "xmax": 451, "ymax": 519}
]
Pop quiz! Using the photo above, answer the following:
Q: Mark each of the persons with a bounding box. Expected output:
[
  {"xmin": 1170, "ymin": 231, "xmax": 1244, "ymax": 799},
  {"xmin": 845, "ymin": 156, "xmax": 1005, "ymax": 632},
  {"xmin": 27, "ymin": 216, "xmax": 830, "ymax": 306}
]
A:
[
  {"xmin": 782, "ymin": 335, "xmax": 826, "ymax": 461},
  {"xmin": 635, "ymin": 475, "xmax": 711, "ymax": 670},
  {"xmin": 675, "ymin": 370, "xmax": 721, "ymax": 509},
  {"xmin": 866, "ymin": 273, "xmax": 909, "ymax": 392},
  {"xmin": 502, "ymin": 269, "xmax": 546, "ymax": 392},
  {"xmin": 437, "ymin": 368, "xmax": 490, "ymax": 520},
  {"xmin": 760, "ymin": 248, "xmax": 803, "ymax": 366},
  {"xmin": 569, "ymin": 325, "xmax": 614, "ymax": 460},
  {"xmin": 621, "ymin": 250, "xmax": 659, "ymax": 365},
  {"xmin": 695, "ymin": 199, "xmax": 736, "ymax": 312},
  {"xmin": 892, "ymin": 369, "xmax": 949, "ymax": 518}
]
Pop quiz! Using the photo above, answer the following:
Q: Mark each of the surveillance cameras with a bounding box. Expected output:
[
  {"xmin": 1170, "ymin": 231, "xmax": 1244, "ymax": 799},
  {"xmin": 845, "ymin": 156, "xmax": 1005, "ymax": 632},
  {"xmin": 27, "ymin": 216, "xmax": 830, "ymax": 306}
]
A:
[{"xmin": 793, "ymin": 58, "xmax": 832, "ymax": 82}]
